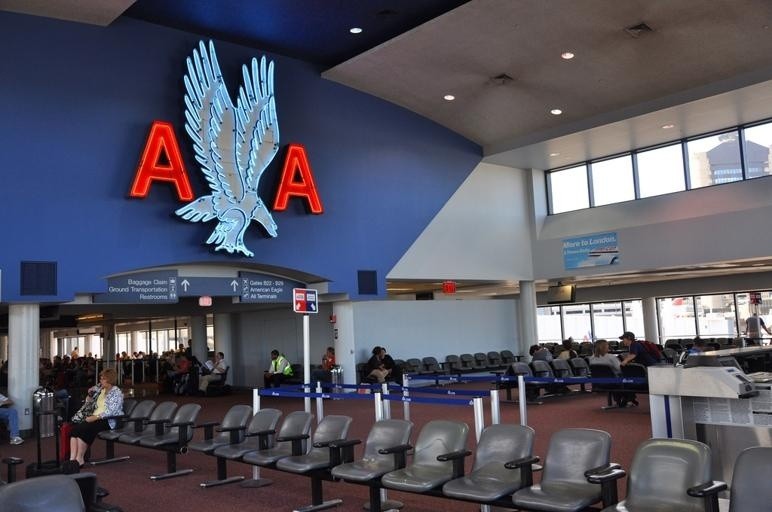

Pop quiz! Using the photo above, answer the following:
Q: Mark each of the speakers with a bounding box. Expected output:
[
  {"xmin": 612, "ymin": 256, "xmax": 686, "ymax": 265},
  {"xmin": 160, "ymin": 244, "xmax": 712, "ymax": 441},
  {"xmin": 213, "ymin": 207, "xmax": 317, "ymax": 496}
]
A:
[
  {"xmin": 19, "ymin": 259, "xmax": 57, "ymax": 295},
  {"xmin": 359, "ymin": 270, "xmax": 377, "ymax": 294}
]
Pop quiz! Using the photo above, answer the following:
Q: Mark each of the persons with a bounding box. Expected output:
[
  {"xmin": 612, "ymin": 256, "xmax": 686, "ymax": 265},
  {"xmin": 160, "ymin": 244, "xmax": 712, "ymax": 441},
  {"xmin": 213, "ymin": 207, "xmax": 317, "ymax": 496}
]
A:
[
  {"xmin": 590, "ymin": 340, "xmax": 638, "ymax": 408},
  {"xmin": 265, "ymin": 349, "xmax": 294, "ymax": 400},
  {"xmin": 317, "ymin": 347, "xmax": 335, "ymax": 382},
  {"xmin": 0, "ymin": 393, "xmax": 25, "ymax": 445},
  {"xmin": 528, "ymin": 344, "xmax": 554, "ymax": 376},
  {"xmin": 376, "ymin": 347, "xmax": 396, "ymax": 375},
  {"xmin": 744, "ymin": 312, "xmax": 772, "ymax": 345},
  {"xmin": 366, "ymin": 347, "xmax": 390, "ymax": 382},
  {"xmin": 556, "ymin": 340, "xmax": 578, "ymax": 376},
  {"xmin": 40, "ymin": 338, "xmax": 228, "ymax": 396},
  {"xmin": 68, "ymin": 368, "xmax": 125, "ymax": 467},
  {"xmin": 618, "ymin": 332, "xmax": 657, "ymax": 366}
]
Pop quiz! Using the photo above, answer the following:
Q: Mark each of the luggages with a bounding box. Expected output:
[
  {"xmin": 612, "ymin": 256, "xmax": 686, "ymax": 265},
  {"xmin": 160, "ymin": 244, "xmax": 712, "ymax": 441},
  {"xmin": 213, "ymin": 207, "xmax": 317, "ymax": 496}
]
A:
[
  {"xmin": 27, "ymin": 410, "xmax": 79, "ymax": 478},
  {"xmin": 59, "ymin": 395, "xmax": 91, "ymax": 461}
]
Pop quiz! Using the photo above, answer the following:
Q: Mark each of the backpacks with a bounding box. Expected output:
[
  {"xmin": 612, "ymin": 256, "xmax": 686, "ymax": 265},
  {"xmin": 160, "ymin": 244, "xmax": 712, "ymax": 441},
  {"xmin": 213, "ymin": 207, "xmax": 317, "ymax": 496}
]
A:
[{"xmin": 638, "ymin": 341, "xmax": 661, "ymax": 360}]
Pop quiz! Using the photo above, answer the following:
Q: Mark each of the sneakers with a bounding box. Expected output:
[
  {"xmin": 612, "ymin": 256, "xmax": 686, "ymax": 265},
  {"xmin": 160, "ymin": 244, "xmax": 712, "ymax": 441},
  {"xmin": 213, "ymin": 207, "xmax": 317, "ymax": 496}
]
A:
[{"xmin": 10, "ymin": 437, "xmax": 24, "ymax": 444}]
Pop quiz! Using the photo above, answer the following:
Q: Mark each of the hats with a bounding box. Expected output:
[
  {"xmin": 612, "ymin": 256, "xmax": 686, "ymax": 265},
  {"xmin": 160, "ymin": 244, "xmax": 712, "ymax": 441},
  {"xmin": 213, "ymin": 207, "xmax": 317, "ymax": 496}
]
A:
[{"xmin": 619, "ymin": 332, "xmax": 635, "ymax": 340}]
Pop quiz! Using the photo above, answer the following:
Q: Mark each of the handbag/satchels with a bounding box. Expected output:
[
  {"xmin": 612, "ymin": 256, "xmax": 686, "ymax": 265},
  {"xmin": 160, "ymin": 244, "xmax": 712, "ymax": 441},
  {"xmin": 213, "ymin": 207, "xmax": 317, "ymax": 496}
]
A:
[{"xmin": 72, "ymin": 401, "xmax": 95, "ymax": 424}]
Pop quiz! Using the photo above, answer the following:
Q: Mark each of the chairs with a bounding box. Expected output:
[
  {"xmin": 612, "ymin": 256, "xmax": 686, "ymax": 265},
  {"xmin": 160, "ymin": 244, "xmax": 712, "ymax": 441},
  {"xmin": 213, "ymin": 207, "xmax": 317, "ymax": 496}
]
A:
[
  {"xmin": 393, "ymin": 350, "xmax": 516, "ymax": 378},
  {"xmin": 590, "ymin": 364, "xmax": 625, "ymax": 410},
  {"xmin": 69, "ymin": 472, "xmax": 110, "ymax": 505},
  {"xmin": 621, "ymin": 362, "xmax": 649, "ymax": 409},
  {"xmin": 357, "ymin": 363, "xmax": 385, "ymax": 396},
  {"xmin": 331, "ymin": 419, "xmax": 772, "ymax": 512},
  {"xmin": 189, "ymin": 404, "xmax": 353, "ymax": 511},
  {"xmin": 206, "ymin": 364, "xmax": 231, "ymax": 397},
  {"xmin": 656, "ymin": 336, "xmax": 755, "ymax": 352},
  {"xmin": 281, "ymin": 364, "xmax": 323, "ymax": 387},
  {"xmin": 606, "ymin": 341, "xmax": 630, "ymax": 353},
  {"xmin": 489, "ymin": 342, "xmax": 593, "ymax": 398},
  {"xmin": 265, "ymin": 335, "xmax": 772, "ymax": 403},
  {"xmin": 0, "ymin": 474, "xmax": 122, "ymax": 511},
  {"xmin": 84, "ymin": 399, "xmax": 771, "ymax": 512},
  {"xmin": 90, "ymin": 400, "xmax": 201, "ymax": 481}
]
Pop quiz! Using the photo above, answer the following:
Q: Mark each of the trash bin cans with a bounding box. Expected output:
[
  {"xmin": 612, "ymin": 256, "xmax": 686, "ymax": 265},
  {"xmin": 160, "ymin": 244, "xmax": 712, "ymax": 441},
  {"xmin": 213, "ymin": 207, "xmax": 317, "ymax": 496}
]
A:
[
  {"xmin": 33, "ymin": 387, "xmax": 55, "ymax": 438},
  {"xmin": 328, "ymin": 364, "xmax": 345, "ymax": 401}
]
